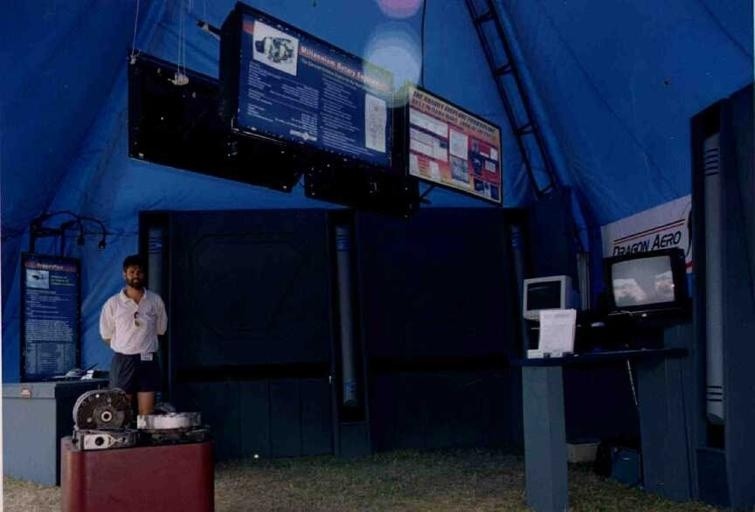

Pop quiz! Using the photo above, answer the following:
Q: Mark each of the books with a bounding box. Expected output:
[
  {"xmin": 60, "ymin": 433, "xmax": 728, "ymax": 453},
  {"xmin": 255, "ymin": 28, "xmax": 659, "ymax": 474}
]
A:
[{"xmin": 526, "ymin": 308, "xmax": 580, "ymax": 360}]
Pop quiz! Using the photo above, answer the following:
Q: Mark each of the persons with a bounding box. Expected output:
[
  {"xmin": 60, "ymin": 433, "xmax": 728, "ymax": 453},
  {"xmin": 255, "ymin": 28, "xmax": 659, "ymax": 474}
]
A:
[{"xmin": 99, "ymin": 255, "xmax": 168, "ymax": 415}]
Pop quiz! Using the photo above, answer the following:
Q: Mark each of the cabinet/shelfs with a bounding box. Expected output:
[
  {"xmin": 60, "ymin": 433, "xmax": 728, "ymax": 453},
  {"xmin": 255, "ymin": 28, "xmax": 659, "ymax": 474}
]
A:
[{"xmin": 3, "ymin": 373, "xmax": 124, "ymax": 490}]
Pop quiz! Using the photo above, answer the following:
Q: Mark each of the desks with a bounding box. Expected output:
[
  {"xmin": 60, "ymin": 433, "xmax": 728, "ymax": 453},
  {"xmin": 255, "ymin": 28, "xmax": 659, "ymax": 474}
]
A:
[{"xmin": 508, "ymin": 345, "xmax": 705, "ymax": 512}]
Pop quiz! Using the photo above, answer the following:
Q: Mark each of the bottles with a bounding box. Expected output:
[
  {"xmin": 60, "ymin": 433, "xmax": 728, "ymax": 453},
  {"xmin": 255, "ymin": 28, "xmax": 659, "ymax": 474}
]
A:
[
  {"xmin": 255, "ymin": 36, "xmax": 294, "ymax": 63},
  {"xmin": 488, "ymin": 146, "xmax": 499, "ymax": 200},
  {"xmin": 470, "ymin": 140, "xmax": 485, "ymax": 194}
]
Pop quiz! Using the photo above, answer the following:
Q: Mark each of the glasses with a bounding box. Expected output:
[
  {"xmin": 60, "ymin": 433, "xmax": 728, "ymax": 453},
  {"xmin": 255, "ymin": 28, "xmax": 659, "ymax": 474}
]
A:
[{"xmin": 134, "ymin": 311, "xmax": 141, "ymax": 327}]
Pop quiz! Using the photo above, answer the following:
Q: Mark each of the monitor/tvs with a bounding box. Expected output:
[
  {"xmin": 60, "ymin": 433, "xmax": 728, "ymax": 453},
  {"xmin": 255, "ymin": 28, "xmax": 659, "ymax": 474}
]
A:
[
  {"xmin": 522, "ymin": 275, "xmax": 571, "ymax": 325},
  {"xmin": 217, "ymin": 1, "xmax": 397, "ymax": 171},
  {"xmin": 302, "ymin": 152, "xmax": 426, "ymax": 223},
  {"xmin": 122, "ymin": 42, "xmax": 303, "ymax": 194},
  {"xmin": 395, "ymin": 76, "xmax": 505, "ymax": 207},
  {"xmin": 601, "ymin": 246, "xmax": 688, "ymax": 311}
]
